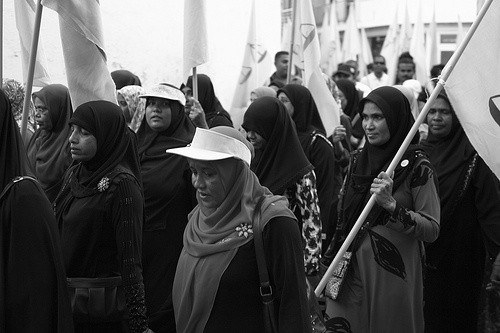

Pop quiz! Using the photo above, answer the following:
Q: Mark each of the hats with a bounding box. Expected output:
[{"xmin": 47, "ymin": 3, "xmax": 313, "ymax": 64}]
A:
[
  {"xmin": 139, "ymin": 85, "xmax": 187, "ymax": 107},
  {"xmin": 335, "ymin": 63, "xmax": 357, "ymax": 76},
  {"xmin": 165, "ymin": 127, "xmax": 252, "ymax": 169}
]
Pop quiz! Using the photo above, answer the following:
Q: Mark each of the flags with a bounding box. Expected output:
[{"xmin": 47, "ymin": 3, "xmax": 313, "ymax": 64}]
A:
[
  {"xmin": 229, "ymin": 0, "xmax": 259, "ymax": 132},
  {"xmin": 13, "ymin": 0, "xmax": 121, "ymax": 115},
  {"xmin": 278, "ymin": 0, "xmax": 500, "ymax": 182},
  {"xmin": 181, "ymin": 0, "xmax": 210, "ymax": 83}
]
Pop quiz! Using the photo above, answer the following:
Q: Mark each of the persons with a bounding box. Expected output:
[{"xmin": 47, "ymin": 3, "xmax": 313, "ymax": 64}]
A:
[
  {"xmin": 250, "ymin": 87, "xmax": 278, "ymax": 104},
  {"xmin": 27, "ymin": 85, "xmax": 74, "ymax": 208},
  {"xmin": 0, "ymin": 90, "xmax": 73, "ymax": 333},
  {"xmin": 165, "ymin": 126, "xmax": 312, "ymax": 333},
  {"xmin": 111, "ymin": 70, "xmax": 143, "ymax": 90},
  {"xmin": 276, "ymin": 84, "xmax": 341, "ymax": 259},
  {"xmin": 326, "ymin": 52, "xmax": 446, "ymax": 153},
  {"xmin": 263, "ymin": 50, "xmax": 305, "ymax": 91},
  {"xmin": 419, "ymin": 88, "xmax": 476, "ymax": 206},
  {"xmin": 136, "ymin": 82, "xmax": 209, "ymax": 333},
  {"xmin": 180, "ymin": 74, "xmax": 236, "ymax": 130},
  {"xmin": 51, "ymin": 99, "xmax": 154, "ymax": 333},
  {"xmin": 2, "ymin": 81, "xmax": 40, "ymax": 150},
  {"xmin": 117, "ymin": 84, "xmax": 148, "ymax": 134},
  {"xmin": 322, "ymin": 87, "xmax": 441, "ymax": 333},
  {"xmin": 240, "ymin": 96, "xmax": 323, "ymax": 333}
]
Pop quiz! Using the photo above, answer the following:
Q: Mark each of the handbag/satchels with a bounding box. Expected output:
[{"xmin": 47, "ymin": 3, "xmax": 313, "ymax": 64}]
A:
[
  {"xmin": 260, "ymin": 276, "xmax": 327, "ymax": 333},
  {"xmin": 319, "ymin": 234, "xmax": 360, "ymax": 300}
]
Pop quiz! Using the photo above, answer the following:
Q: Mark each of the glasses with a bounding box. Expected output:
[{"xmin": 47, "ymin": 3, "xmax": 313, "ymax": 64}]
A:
[{"xmin": 375, "ymin": 62, "xmax": 384, "ymax": 65}]
